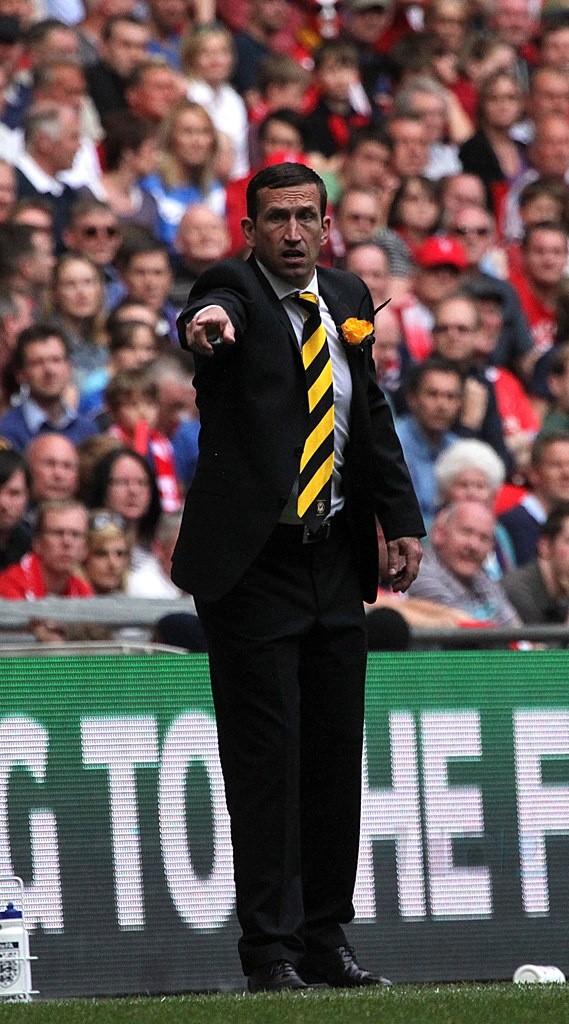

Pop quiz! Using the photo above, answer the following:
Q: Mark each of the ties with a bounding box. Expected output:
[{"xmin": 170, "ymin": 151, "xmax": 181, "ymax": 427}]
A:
[{"xmin": 286, "ymin": 292, "xmax": 335, "ymax": 535}]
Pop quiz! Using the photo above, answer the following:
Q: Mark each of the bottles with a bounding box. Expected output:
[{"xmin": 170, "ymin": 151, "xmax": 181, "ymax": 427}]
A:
[
  {"xmin": 513, "ymin": 965, "xmax": 566, "ymax": 984},
  {"xmin": 0, "ymin": 903, "xmax": 31, "ymax": 1002}
]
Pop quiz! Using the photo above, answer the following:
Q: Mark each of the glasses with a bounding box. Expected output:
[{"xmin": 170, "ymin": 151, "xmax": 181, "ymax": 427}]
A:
[
  {"xmin": 434, "ymin": 323, "xmax": 475, "ymax": 333},
  {"xmin": 88, "ymin": 511, "xmax": 127, "ymax": 533},
  {"xmin": 452, "ymin": 225, "xmax": 491, "ymax": 238},
  {"xmin": 69, "ymin": 225, "xmax": 118, "ymax": 238}
]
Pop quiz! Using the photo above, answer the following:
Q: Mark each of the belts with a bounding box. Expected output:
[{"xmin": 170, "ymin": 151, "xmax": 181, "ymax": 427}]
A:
[{"xmin": 271, "ymin": 509, "xmax": 345, "ymax": 545}]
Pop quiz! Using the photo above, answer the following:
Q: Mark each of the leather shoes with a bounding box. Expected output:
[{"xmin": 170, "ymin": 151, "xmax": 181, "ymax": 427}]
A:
[
  {"xmin": 247, "ymin": 959, "xmax": 329, "ymax": 994},
  {"xmin": 311, "ymin": 945, "xmax": 394, "ymax": 988}
]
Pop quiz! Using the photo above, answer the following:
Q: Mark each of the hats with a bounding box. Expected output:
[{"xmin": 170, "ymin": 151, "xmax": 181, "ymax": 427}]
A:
[{"xmin": 413, "ymin": 235, "xmax": 466, "ymax": 272}]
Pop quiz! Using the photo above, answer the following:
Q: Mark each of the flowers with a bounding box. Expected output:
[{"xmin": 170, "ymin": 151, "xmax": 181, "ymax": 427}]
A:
[{"xmin": 341, "ymin": 317, "xmax": 373, "ymax": 344}]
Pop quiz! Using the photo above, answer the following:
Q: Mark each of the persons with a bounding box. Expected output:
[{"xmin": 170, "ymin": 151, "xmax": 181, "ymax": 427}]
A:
[
  {"xmin": 170, "ymin": 163, "xmax": 428, "ymax": 993},
  {"xmin": 0, "ymin": 0, "xmax": 569, "ymax": 650}
]
